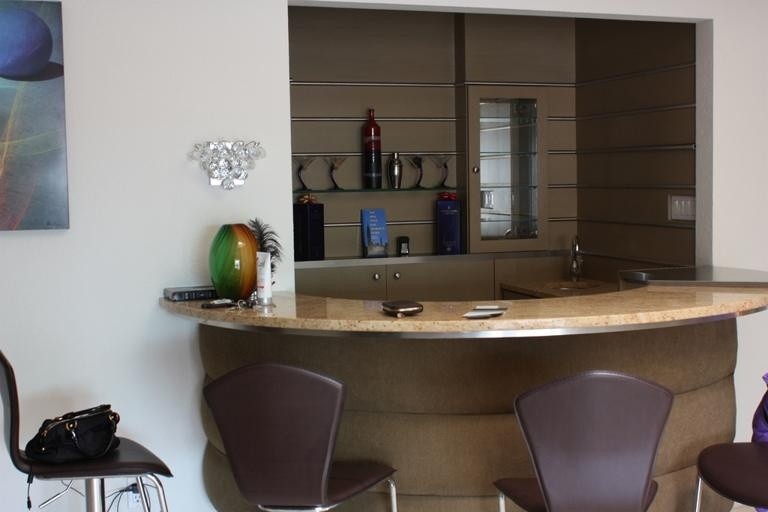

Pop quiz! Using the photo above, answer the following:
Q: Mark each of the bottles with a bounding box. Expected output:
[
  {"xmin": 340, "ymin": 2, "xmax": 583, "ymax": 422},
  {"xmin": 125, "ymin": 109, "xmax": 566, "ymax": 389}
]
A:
[
  {"xmin": 360, "ymin": 108, "xmax": 381, "ymax": 189},
  {"xmin": 389, "ymin": 152, "xmax": 403, "ymax": 189}
]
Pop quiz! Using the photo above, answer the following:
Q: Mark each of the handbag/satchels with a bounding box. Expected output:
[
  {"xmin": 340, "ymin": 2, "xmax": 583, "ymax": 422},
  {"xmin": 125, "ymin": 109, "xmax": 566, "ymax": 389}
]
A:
[{"xmin": 24, "ymin": 404, "xmax": 120, "ymax": 464}]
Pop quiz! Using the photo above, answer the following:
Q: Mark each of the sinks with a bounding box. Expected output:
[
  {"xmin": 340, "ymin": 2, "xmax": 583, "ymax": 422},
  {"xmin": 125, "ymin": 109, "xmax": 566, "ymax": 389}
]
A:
[{"xmin": 556, "ymin": 279, "xmax": 603, "ymax": 292}]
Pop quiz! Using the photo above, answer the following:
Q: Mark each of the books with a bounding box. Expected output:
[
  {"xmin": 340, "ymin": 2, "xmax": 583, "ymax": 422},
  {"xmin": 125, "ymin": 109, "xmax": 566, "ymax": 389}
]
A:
[{"xmin": 383, "ymin": 299, "xmax": 424, "ymax": 317}]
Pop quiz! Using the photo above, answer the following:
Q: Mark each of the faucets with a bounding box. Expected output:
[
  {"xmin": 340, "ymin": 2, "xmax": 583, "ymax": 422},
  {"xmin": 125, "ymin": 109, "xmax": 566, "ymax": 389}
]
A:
[{"xmin": 570, "ymin": 239, "xmax": 580, "ymax": 282}]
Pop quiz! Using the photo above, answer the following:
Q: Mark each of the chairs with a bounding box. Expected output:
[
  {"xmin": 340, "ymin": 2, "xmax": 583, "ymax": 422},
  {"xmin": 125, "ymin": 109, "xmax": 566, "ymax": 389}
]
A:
[
  {"xmin": 203, "ymin": 361, "xmax": 400, "ymax": 512},
  {"xmin": 0, "ymin": 352, "xmax": 172, "ymax": 511},
  {"xmin": 491, "ymin": 371, "xmax": 674, "ymax": 512}
]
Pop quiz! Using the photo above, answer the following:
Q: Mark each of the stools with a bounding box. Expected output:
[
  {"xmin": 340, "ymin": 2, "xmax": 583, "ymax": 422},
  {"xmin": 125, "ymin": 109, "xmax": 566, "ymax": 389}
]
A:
[{"xmin": 693, "ymin": 437, "xmax": 766, "ymax": 512}]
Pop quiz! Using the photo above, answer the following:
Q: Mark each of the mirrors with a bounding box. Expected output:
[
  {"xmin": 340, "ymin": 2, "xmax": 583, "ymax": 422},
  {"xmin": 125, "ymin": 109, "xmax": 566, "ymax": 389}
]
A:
[{"xmin": 476, "ymin": 92, "xmax": 541, "ymax": 242}]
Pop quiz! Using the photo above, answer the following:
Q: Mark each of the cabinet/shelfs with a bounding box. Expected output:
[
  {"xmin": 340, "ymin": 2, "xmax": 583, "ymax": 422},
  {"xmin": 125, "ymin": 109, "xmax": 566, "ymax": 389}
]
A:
[
  {"xmin": 294, "ymin": 258, "xmax": 495, "ymax": 306},
  {"xmin": 459, "ymin": 85, "xmax": 550, "ymax": 251}
]
